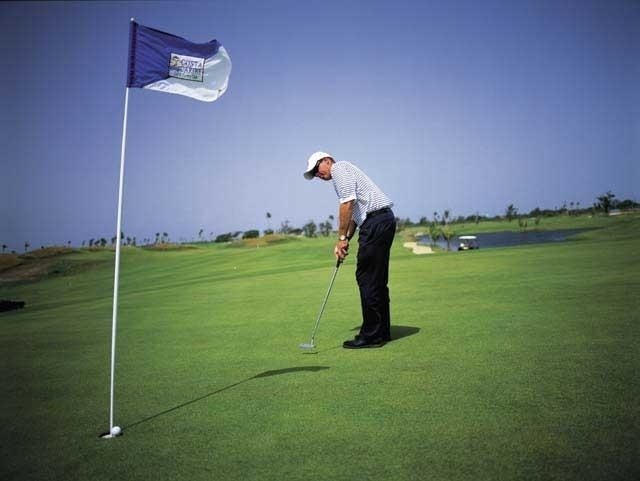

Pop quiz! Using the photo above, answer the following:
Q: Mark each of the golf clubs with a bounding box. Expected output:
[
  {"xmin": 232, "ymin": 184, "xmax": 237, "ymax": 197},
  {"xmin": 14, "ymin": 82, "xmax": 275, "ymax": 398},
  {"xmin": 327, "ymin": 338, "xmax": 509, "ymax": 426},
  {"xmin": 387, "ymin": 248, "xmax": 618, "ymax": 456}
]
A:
[{"xmin": 298, "ymin": 255, "xmax": 341, "ymax": 349}]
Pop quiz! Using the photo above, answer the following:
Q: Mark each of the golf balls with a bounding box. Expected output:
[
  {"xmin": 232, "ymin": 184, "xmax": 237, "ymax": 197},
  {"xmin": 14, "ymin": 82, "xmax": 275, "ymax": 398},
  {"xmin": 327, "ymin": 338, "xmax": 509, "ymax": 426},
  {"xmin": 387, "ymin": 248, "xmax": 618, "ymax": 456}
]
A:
[{"xmin": 111, "ymin": 425, "xmax": 120, "ymax": 434}]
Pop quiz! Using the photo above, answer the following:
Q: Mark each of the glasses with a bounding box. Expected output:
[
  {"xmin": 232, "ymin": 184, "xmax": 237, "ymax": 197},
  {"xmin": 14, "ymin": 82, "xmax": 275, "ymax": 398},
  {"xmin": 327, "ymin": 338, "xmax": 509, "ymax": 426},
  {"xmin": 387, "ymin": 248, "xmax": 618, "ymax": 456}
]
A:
[{"xmin": 312, "ymin": 162, "xmax": 319, "ymax": 177}]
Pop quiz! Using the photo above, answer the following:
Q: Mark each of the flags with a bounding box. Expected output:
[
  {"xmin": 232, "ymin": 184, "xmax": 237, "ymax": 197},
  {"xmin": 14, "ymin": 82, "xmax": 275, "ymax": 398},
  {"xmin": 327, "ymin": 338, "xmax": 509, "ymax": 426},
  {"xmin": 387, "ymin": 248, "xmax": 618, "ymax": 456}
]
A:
[{"xmin": 126, "ymin": 21, "xmax": 232, "ymax": 102}]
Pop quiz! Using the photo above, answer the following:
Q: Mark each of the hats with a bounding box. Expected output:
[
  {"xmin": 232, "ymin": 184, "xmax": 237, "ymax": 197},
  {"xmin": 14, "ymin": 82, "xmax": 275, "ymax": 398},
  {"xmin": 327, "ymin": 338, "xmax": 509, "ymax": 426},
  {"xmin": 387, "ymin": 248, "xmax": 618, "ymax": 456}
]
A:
[{"xmin": 303, "ymin": 152, "xmax": 336, "ymax": 181}]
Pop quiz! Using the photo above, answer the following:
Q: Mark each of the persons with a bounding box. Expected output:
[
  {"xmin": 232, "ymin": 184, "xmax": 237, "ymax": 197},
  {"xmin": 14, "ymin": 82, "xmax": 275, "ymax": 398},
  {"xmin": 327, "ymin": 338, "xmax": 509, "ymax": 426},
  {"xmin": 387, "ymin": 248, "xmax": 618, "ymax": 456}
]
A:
[{"xmin": 303, "ymin": 150, "xmax": 396, "ymax": 349}]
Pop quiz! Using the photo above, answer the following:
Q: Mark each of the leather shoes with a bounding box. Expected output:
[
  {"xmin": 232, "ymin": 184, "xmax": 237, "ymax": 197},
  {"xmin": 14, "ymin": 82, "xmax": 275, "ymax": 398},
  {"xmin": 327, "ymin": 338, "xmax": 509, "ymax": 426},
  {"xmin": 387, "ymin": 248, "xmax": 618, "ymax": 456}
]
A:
[{"xmin": 343, "ymin": 331, "xmax": 393, "ymax": 349}]
